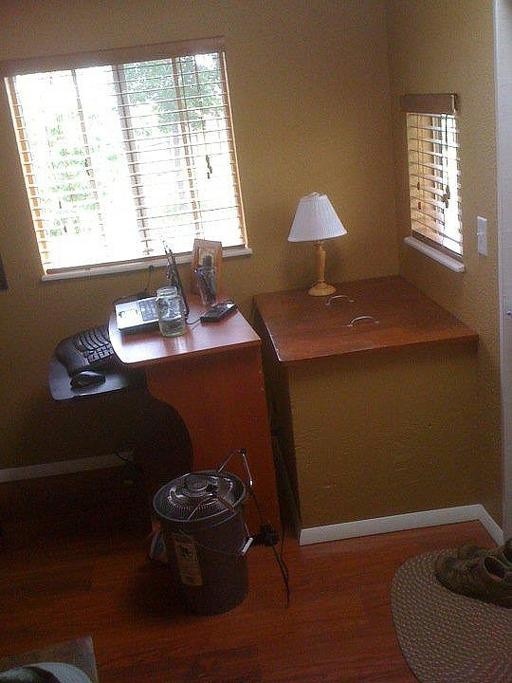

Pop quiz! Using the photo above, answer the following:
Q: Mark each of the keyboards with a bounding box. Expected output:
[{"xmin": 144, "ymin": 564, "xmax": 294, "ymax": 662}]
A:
[{"xmin": 54, "ymin": 325, "xmax": 114, "ymax": 374}]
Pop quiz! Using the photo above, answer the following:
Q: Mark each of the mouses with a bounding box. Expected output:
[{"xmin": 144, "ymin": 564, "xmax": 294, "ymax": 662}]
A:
[{"xmin": 69, "ymin": 364, "xmax": 108, "ymax": 390}]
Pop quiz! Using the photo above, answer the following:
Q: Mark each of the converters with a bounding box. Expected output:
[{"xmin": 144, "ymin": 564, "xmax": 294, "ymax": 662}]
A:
[{"xmin": 199, "ymin": 300, "xmax": 237, "ymax": 323}]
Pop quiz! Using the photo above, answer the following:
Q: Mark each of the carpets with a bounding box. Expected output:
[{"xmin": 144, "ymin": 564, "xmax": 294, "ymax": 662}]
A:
[{"xmin": 391, "ymin": 552, "xmax": 512, "ymax": 682}]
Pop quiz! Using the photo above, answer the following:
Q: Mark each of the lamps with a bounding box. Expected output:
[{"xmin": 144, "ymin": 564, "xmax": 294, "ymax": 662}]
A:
[{"xmin": 288, "ymin": 191, "xmax": 348, "ymax": 297}]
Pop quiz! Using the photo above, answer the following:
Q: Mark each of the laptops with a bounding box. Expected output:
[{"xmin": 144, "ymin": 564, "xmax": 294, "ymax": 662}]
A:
[{"xmin": 114, "ymin": 241, "xmax": 186, "ymax": 334}]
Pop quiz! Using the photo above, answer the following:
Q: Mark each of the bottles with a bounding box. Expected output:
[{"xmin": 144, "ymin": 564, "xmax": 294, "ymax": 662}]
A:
[{"xmin": 155, "ymin": 285, "xmax": 186, "ymax": 337}]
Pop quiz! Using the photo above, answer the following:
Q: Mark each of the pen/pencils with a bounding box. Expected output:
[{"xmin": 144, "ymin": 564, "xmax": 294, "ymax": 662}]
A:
[{"xmin": 194, "ymin": 267, "xmax": 217, "ymax": 305}]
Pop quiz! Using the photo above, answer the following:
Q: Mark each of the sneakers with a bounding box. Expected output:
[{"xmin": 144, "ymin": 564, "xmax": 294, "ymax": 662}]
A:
[{"xmin": 434, "ymin": 538, "xmax": 512, "ymax": 609}]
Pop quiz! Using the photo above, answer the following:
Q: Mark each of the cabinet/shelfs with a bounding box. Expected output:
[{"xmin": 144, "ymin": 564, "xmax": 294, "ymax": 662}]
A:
[
  {"xmin": 46, "ymin": 296, "xmax": 283, "ymax": 546},
  {"xmin": 254, "ymin": 275, "xmax": 482, "ymax": 547}
]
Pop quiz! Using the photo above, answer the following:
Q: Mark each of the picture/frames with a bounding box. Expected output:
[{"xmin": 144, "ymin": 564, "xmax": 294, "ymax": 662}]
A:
[{"xmin": 191, "ymin": 238, "xmax": 223, "ymax": 298}]
